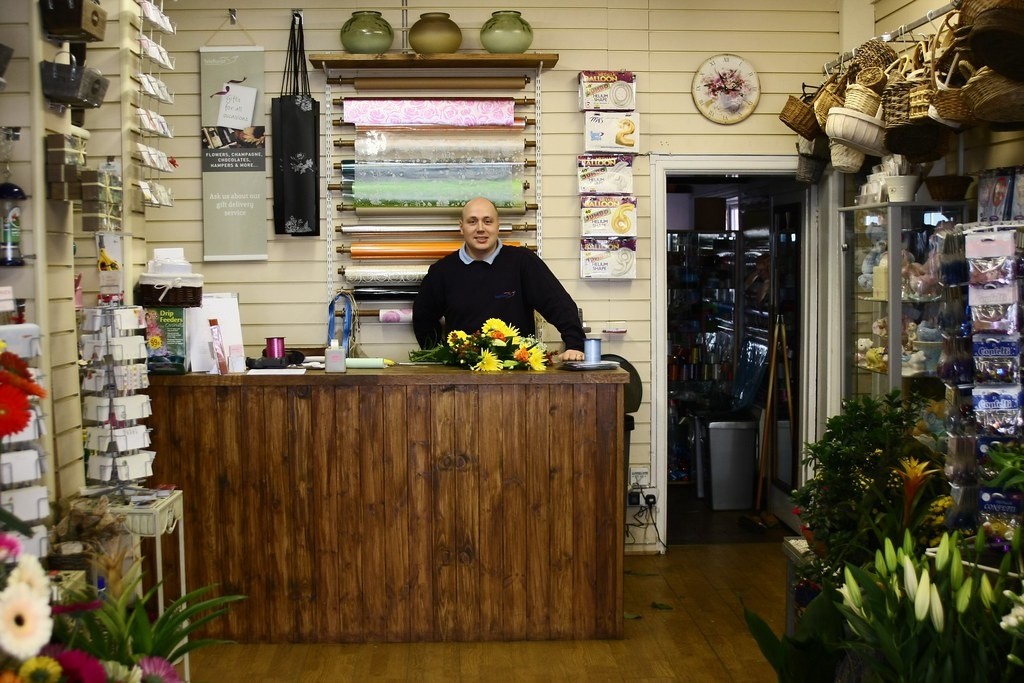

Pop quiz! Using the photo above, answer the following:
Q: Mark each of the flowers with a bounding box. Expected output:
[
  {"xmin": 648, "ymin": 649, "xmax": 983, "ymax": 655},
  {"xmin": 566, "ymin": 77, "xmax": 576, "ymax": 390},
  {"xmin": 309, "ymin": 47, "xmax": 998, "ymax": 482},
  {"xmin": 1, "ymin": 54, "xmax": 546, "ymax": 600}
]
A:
[
  {"xmin": 0, "ymin": 340, "xmax": 248, "ymax": 683},
  {"xmin": 409, "ymin": 316, "xmax": 560, "ymax": 373},
  {"xmin": 834, "ymin": 455, "xmax": 1024, "ymax": 683}
]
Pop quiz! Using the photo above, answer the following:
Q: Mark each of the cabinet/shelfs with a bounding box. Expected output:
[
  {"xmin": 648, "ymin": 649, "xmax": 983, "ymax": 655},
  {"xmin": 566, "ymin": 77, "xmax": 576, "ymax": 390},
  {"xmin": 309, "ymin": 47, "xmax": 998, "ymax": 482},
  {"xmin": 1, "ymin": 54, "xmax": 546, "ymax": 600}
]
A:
[
  {"xmin": 833, "ymin": 198, "xmax": 973, "ymax": 462},
  {"xmin": 661, "ymin": 178, "xmax": 804, "ymax": 457}
]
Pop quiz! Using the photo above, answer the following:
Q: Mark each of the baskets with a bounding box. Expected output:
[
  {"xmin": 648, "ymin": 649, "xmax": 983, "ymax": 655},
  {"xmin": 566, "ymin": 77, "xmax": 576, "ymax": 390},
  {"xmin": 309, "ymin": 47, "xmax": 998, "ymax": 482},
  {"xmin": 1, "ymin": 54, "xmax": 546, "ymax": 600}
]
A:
[{"xmin": 779, "ymin": 0, "xmax": 1024, "ymax": 185}]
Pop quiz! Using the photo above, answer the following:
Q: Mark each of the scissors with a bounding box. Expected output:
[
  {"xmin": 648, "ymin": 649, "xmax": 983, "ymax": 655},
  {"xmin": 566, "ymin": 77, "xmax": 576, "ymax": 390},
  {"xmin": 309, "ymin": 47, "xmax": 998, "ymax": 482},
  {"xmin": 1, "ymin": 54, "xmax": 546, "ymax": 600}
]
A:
[{"xmin": 98, "ymin": 235, "xmax": 119, "ymax": 271}]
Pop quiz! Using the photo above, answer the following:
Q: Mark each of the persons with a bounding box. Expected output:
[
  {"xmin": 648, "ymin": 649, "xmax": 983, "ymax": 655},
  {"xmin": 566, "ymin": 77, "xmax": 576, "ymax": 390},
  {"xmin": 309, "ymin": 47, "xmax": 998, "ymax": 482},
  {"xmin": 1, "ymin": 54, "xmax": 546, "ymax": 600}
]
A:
[{"xmin": 413, "ymin": 198, "xmax": 586, "ymax": 361}]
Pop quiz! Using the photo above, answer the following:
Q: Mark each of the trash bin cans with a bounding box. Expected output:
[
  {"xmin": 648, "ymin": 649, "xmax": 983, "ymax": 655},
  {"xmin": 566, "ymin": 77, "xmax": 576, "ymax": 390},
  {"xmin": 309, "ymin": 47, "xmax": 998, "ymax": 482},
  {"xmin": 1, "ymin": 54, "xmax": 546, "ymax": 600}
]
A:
[{"xmin": 706, "ymin": 415, "xmax": 756, "ymax": 510}]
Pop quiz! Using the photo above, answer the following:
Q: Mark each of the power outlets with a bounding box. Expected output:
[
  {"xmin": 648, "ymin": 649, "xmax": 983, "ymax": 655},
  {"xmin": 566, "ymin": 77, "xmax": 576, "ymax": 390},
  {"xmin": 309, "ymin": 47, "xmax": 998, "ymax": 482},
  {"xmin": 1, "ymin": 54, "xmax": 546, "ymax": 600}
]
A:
[{"xmin": 629, "ymin": 467, "xmax": 649, "ymax": 487}]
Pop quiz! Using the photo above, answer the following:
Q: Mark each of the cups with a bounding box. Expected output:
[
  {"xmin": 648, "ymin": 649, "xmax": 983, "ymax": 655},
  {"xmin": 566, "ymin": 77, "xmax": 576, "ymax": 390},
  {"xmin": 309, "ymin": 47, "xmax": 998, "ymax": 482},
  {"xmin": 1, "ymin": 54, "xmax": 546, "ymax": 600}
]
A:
[{"xmin": 584, "ymin": 339, "xmax": 601, "ymax": 363}]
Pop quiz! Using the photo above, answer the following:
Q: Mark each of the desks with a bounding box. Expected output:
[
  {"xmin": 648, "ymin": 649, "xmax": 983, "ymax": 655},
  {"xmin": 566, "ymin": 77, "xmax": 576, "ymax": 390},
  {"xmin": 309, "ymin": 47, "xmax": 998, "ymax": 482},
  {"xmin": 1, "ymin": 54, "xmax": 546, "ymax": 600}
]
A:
[{"xmin": 65, "ymin": 487, "xmax": 191, "ymax": 682}]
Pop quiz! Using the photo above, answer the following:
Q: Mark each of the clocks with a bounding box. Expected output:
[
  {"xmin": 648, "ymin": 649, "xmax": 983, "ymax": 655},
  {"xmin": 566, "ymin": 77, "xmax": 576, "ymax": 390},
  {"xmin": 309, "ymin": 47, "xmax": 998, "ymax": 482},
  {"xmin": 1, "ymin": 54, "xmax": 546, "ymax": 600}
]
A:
[{"xmin": 690, "ymin": 53, "xmax": 762, "ymax": 125}]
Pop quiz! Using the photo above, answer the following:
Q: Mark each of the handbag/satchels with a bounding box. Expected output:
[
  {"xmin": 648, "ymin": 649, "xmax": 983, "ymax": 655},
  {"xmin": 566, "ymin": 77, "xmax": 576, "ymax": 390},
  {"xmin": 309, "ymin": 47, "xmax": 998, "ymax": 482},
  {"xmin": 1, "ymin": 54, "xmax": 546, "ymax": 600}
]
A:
[{"xmin": 272, "ymin": 12, "xmax": 320, "ymax": 237}]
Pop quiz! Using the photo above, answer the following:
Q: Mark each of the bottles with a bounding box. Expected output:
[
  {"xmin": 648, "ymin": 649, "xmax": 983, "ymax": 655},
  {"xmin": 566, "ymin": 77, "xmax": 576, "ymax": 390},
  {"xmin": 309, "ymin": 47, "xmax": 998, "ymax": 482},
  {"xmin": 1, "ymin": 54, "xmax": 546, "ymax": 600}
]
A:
[
  {"xmin": 408, "ymin": 12, "xmax": 462, "ymax": 53},
  {"xmin": 209, "ymin": 319, "xmax": 230, "ymax": 376},
  {"xmin": 340, "ymin": 10, "xmax": 394, "ymax": 54},
  {"xmin": 480, "ymin": 10, "xmax": 533, "ymax": 54}
]
